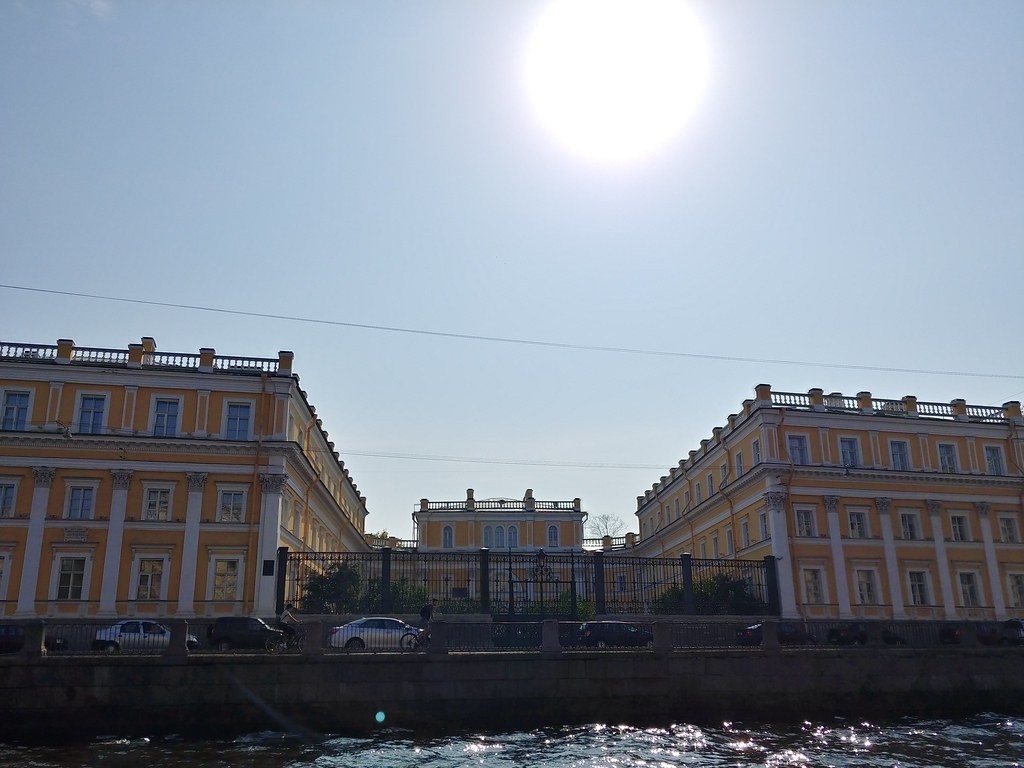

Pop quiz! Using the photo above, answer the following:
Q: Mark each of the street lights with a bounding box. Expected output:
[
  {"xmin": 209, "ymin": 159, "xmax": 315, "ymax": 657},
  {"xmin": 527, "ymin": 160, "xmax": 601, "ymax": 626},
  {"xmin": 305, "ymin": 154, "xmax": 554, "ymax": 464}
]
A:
[{"xmin": 536, "ymin": 548, "xmax": 548, "ymax": 614}]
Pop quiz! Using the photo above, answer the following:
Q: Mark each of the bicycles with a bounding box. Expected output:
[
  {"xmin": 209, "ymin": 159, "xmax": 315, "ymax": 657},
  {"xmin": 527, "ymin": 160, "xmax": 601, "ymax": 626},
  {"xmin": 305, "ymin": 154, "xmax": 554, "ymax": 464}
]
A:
[
  {"xmin": 398, "ymin": 623, "xmax": 457, "ymax": 653},
  {"xmin": 265, "ymin": 619, "xmax": 309, "ymax": 654}
]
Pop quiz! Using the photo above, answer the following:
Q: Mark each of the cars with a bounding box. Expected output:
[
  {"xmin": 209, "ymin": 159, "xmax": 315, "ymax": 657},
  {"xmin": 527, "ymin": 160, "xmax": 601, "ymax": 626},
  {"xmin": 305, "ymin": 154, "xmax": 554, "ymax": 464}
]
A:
[
  {"xmin": 828, "ymin": 623, "xmax": 907, "ymax": 647},
  {"xmin": 976, "ymin": 621, "xmax": 1024, "ymax": 647},
  {"xmin": 94, "ymin": 620, "xmax": 199, "ymax": 654},
  {"xmin": 576, "ymin": 622, "xmax": 653, "ymax": 650},
  {"xmin": 326, "ymin": 617, "xmax": 431, "ymax": 653},
  {"xmin": 939, "ymin": 623, "xmax": 964, "ymax": 645},
  {"xmin": 208, "ymin": 617, "xmax": 287, "ymax": 653},
  {"xmin": 0, "ymin": 625, "xmax": 69, "ymax": 654},
  {"xmin": 742, "ymin": 622, "xmax": 818, "ymax": 645}
]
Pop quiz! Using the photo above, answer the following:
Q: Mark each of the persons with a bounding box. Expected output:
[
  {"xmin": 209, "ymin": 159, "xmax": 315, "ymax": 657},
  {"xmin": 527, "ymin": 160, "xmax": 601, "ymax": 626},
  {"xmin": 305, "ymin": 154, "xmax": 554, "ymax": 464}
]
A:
[
  {"xmin": 278, "ymin": 604, "xmax": 302, "ymax": 648},
  {"xmin": 420, "ymin": 599, "xmax": 438, "ymax": 638}
]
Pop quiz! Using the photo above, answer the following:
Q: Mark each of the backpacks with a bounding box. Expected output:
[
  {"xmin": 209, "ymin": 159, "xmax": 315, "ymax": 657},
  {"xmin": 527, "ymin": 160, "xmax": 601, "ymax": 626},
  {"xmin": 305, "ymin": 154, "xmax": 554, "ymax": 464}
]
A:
[{"xmin": 420, "ymin": 604, "xmax": 432, "ymax": 617}]
